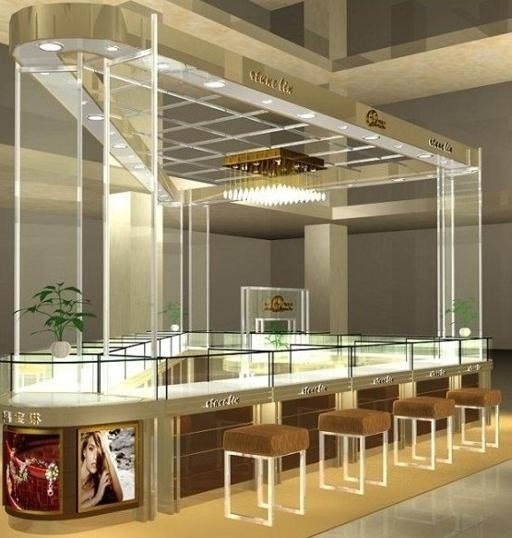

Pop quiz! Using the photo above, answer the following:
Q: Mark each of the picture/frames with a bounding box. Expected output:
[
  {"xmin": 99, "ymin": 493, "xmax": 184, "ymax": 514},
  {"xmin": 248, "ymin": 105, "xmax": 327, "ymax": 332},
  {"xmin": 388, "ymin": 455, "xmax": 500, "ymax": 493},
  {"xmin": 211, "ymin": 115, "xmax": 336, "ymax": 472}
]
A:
[
  {"xmin": 1, "ymin": 424, "xmax": 64, "ymax": 520},
  {"xmin": 74, "ymin": 418, "xmax": 145, "ymax": 515}
]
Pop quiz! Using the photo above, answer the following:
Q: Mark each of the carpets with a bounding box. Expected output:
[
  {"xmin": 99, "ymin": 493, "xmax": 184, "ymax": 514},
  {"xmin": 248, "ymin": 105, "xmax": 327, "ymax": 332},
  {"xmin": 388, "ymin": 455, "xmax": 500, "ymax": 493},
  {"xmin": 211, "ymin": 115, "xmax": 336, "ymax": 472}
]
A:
[{"xmin": 65, "ymin": 414, "xmax": 512, "ymax": 538}]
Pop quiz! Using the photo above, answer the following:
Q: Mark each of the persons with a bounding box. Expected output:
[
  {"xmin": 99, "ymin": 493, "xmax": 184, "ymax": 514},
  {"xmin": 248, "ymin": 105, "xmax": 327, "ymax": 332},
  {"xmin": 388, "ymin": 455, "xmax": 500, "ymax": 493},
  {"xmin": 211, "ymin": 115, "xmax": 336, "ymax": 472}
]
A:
[{"xmin": 78, "ymin": 430, "xmax": 123, "ymax": 511}]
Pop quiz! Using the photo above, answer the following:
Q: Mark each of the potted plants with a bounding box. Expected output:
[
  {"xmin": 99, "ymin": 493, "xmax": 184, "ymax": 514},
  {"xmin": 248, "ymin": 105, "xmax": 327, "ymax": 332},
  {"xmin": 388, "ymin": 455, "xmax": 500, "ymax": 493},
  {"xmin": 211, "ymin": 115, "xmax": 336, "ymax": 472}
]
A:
[
  {"xmin": 11, "ymin": 281, "xmax": 98, "ymax": 359},
  {"xmin": 157, "ymin": 298, "xmax": 189, "ymax": 332},
  {"xmin": 443, "ymin": 295, "xmax": 480, "ymax": 338}
]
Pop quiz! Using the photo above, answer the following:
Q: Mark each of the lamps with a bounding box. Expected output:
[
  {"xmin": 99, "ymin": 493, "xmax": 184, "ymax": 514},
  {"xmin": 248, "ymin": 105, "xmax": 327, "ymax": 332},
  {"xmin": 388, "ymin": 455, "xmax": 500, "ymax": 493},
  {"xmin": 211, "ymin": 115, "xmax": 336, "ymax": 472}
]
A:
[{"xmin": 223, "ymin": 149, "xmax": 327, "ymax": 208}]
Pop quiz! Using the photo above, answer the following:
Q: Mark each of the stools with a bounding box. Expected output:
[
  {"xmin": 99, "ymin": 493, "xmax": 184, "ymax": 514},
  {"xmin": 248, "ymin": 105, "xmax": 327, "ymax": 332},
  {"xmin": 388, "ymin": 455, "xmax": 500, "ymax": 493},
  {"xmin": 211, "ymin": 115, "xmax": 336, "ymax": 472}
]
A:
[
  {"xmin": 445, "ymin": 388, "xmax": 502, "ymax": 454},
  {"xmin": 222, "ymin": 423, "xmax": 310, "ymax": 529},
  {"xmin": 392, "ymin": 396, "xmax": 455, "ymax": 470},
  {"xmin": 317, "ymin": 408, "xmax": 390, "ymax": 496}
]
